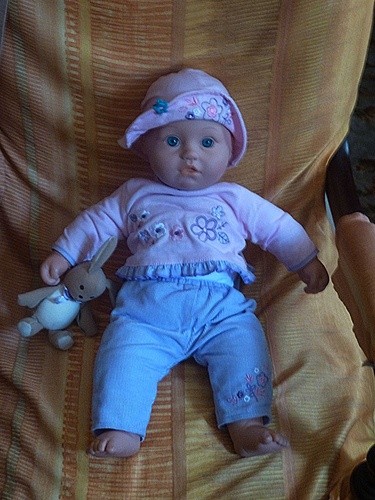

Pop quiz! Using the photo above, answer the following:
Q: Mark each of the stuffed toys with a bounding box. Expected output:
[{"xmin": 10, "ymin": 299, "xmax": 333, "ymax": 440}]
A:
[{"xmin": 18, "ymin": 237, "xmax": 118, "ymax": 352}]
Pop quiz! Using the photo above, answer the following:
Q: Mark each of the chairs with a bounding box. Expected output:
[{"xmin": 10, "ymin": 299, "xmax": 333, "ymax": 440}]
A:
[{"xmin": 1, "ymin": 0, "xmax": 374, "ymax": 500}]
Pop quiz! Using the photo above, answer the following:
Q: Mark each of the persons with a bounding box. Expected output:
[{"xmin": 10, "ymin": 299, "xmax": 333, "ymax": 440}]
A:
[{"xmin": 40, "ymin": 71, "xmax": 329, "ymax": 461}]
